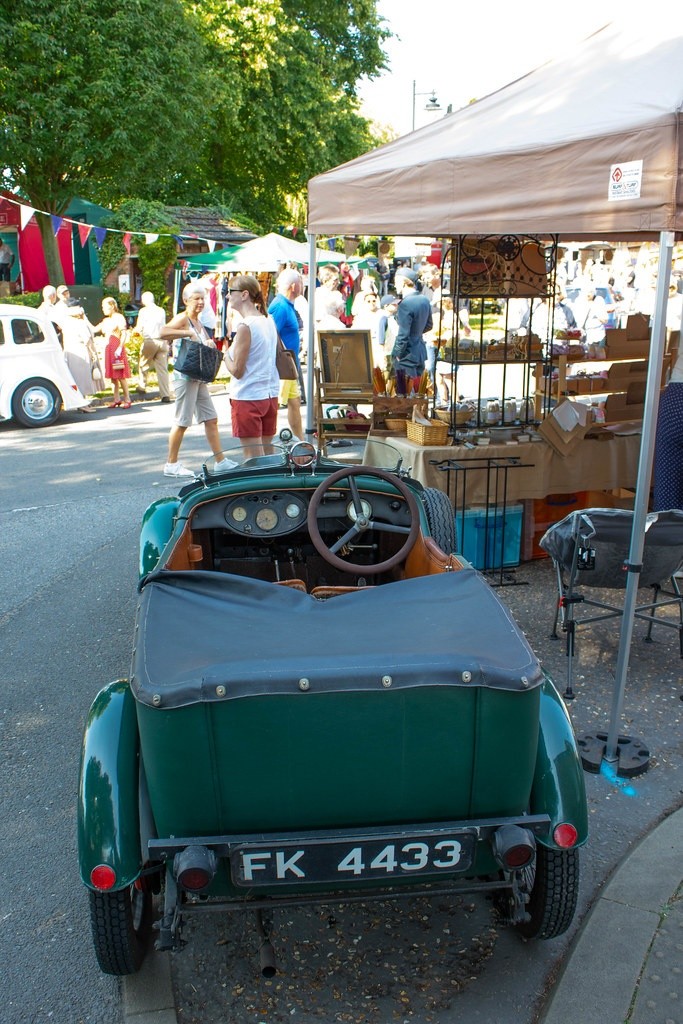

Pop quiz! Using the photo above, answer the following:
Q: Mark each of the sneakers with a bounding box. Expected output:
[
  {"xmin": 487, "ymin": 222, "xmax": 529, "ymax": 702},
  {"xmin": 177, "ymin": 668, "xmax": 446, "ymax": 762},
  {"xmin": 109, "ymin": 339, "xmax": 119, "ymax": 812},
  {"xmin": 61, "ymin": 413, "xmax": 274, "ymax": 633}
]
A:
[
  {"xmin": 163, "ymin": 463, "xmax": 194, "ymax": 478},
  {"xmin": 214, "ymin": 458, "xmax": 239, "ymax": 472}
]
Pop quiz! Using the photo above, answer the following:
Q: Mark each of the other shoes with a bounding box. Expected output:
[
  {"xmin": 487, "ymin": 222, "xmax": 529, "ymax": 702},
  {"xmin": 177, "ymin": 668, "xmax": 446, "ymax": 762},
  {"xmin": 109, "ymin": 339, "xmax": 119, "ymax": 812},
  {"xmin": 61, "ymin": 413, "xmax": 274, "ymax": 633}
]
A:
[
  {"xmin": 162, "ymin": 396, "xmax": 170, "ymax": 402},
  {"xmin": 136, "ymin": 386, "xmax": 146, "ymax": 394},
  {"xmin": 79, "ymin": 408, "xmax": 96, "ymax": 414}
]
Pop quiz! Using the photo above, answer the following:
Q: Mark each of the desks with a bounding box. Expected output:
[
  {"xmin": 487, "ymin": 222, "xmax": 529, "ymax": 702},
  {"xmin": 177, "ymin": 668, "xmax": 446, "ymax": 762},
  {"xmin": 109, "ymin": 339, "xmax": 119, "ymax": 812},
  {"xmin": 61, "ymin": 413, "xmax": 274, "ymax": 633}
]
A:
[{"xmin": 359, "ymin": 422, "xmax": 654, "ymax": 504}]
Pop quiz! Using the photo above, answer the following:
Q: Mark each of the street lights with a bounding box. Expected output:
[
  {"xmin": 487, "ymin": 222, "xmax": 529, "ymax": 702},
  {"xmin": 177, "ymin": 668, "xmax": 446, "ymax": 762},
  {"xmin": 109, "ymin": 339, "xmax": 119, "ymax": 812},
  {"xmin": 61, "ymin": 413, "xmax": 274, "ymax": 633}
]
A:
[{"xmin": 411, "ymin": 79, "xmax": 443, "ymax": 133}]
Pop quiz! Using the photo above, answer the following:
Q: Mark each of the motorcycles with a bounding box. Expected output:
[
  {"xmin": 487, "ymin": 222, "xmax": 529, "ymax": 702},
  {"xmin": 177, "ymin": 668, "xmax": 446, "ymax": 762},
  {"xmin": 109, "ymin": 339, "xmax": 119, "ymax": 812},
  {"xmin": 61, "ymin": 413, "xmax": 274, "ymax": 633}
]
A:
[{"xmin": 75, "ymin": 429, "xmax": 591, "ymax": 978}]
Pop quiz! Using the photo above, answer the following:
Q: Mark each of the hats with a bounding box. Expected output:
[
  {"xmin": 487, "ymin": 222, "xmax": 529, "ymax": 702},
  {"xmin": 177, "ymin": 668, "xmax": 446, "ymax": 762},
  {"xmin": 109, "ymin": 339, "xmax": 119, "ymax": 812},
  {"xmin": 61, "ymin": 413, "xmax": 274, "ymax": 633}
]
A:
[
  {"xmin": 57, "ymin": 285, "xmax": 69, "ymax": 300},
  {"xmin": 396, "ymin": 268, "xmax": 419, "ymax": 282},
  {"xmin": 381, "ymin": 294, "xmax": 401, "ymax": 309}
]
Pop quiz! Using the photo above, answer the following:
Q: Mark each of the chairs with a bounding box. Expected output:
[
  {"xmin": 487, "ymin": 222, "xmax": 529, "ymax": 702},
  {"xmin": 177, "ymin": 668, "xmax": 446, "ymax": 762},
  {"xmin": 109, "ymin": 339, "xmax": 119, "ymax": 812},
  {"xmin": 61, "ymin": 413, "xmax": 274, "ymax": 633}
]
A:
[{"xmin": 538, "ymin": 508, "xmax": 683, "ymax": 699}]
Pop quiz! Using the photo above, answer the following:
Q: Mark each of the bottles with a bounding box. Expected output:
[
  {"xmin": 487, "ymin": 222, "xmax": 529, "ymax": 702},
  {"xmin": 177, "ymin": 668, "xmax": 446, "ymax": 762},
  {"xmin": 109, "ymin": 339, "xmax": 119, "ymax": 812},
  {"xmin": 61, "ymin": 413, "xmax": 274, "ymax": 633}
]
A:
[
  {"xmin": 485, "ymin": 397, "xmax": 535, "ymax": 426},
  {"xmin": 590, "ymin": 402, "xmax": 599, "ymax": 422},
  {"xmin": 588, "ymin": 341, "xmax": 601, "ymax": 359},
  {"xmin": 577, "ymin": 370, "xmax": 588, "ymax": 380}
]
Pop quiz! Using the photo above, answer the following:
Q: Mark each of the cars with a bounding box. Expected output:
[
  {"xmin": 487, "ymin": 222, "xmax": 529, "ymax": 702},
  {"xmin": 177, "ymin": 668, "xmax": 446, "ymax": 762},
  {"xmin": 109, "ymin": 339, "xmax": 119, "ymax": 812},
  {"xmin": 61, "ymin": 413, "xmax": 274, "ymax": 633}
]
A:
[{"xmin": 0, "ymin": 302, "xmax": 106, "ymax": 430}]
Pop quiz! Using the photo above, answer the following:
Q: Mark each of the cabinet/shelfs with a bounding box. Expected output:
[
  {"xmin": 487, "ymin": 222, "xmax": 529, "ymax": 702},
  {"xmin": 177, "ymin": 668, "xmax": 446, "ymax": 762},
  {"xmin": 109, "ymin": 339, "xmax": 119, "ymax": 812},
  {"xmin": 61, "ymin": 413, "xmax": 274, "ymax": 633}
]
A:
[
  {"xmin": 434, "ymin": 233, "xmax": 558, "ymax": 433},
  {"xmin": 533, "ymin": 348, "xmax": 678, "ymax": 427}
]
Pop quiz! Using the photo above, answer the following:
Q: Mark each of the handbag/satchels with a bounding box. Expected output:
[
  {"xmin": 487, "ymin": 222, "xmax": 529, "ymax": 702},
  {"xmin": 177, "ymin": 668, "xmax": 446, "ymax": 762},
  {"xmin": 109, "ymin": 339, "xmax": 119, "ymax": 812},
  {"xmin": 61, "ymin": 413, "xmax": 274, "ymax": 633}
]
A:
[
  {"xmin": 276, "ymin": 332, "xmax": 300, "ymax": 379},
  {"xmin": 174, "ymin": 317, "xmax": 224, "ymax": 383},
  {"xmin": 580, "ymin": 325, "xmax": 586, "ymax": 343},
  {"xmin": 113, "ymin": 357, "xmax": 125, "ymax": 369},
  {"xmin": 92, "ymin": 363, "xmax": 101, "ymax": 380}
]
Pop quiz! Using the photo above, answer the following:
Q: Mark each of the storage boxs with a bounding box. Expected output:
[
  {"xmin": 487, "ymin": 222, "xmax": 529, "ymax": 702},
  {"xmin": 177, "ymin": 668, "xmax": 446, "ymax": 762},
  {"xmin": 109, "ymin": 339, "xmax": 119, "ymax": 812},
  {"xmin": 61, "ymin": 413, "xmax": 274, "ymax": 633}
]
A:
[
  {"xmin": 452, "ymin": 501, "xmax": 524, "ymax": 569},
  {"xmin": 586, "ymin": 488, "xmax": 636, "ymax": 511},
  {"xmin": 538, "ymin": 375, "xmax": 604, "ymax": 395}
]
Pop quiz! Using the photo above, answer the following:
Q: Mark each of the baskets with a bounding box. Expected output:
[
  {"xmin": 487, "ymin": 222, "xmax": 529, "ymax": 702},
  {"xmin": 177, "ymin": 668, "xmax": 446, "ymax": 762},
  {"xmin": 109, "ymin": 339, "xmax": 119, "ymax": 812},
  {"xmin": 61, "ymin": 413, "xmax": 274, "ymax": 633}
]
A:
[
  {"xmin": 434, "ymin": 406, "xmax": 473, "ymax": 425},
  {"xmin": 385, "ymin": 418, "xmax": 409, "ymax": 430},
  {"xmin": 405, "ymin": 418, "xmax": 450, "ymax": 446}
]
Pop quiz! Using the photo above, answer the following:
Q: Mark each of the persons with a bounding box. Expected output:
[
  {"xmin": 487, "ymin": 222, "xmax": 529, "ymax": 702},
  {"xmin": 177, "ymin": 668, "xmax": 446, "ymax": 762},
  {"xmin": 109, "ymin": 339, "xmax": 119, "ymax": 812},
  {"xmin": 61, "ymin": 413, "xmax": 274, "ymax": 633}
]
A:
[
  {"xmin": 269, "ymin": 270, "xmax": 305, "ymax": 443},
  {"xmin": 223, "ymin": 277, "xmax": 284, "ymax": 462},
  {"xmin": 392, "ymin": 267, "xmax": 434, "ymax": 380},
  {"xmin": 160, "ymin": 283, "xmax": 239, "ymax": 480},
  {"xmin": 655, "ymin": 352, "xmax": 683, "ymax": 511},
  {"xmin": 37, "ymin": 252, "xmax": 683, "ymax": 412}
]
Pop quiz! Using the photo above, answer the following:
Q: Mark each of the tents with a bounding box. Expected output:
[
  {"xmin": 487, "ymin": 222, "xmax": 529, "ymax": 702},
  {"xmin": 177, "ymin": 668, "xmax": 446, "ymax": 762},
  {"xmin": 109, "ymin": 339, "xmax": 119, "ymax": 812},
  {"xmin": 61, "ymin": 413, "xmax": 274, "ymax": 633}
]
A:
[
  {"xmin": 307, "ymin": 0, "xmax": 683, "ymax": 778},
  {"xmin": 172, "ymin": 232, "xmax": 368, "ymax": 317}
]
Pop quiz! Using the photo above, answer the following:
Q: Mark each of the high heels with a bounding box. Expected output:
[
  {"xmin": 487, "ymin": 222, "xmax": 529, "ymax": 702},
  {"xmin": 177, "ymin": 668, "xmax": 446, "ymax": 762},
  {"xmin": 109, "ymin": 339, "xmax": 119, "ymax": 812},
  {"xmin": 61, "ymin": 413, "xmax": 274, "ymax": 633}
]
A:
[
  {"xmin": 123, "ymin": 401, "xmax": 131, "ymax": 409},
  {"xmin": 107, "ymin": 399, "xmax": 122, "ymax": 408}
]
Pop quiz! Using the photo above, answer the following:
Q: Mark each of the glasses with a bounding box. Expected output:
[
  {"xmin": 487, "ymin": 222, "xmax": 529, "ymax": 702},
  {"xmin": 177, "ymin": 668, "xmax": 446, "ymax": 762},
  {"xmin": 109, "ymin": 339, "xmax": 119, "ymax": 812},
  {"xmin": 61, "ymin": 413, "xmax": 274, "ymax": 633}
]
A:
[{"xmin": 227, "ymin": 289, "xmax": 243, "ymax": 295}]
